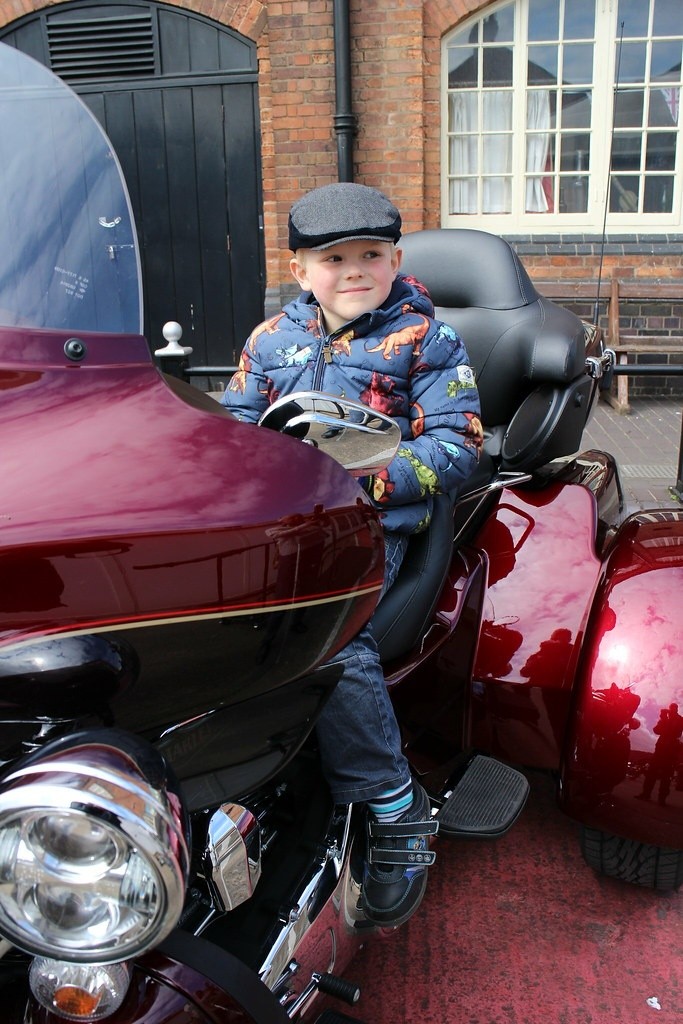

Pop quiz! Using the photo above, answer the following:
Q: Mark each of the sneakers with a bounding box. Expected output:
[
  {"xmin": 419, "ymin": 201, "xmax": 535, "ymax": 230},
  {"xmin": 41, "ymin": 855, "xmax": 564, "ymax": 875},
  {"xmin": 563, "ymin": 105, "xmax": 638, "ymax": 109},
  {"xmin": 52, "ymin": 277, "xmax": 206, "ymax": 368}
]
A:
[{"xmin": 360, "ymin": 779, "xmax": 440, "ymax": 927}]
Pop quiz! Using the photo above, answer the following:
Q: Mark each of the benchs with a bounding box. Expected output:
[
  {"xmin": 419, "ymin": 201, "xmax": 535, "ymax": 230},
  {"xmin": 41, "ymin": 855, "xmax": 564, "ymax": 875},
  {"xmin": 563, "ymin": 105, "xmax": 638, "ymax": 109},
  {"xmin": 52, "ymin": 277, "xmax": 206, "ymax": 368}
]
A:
[{"xmin": 604, "ymin": 280, "xmax": 683, "ymax": 416}]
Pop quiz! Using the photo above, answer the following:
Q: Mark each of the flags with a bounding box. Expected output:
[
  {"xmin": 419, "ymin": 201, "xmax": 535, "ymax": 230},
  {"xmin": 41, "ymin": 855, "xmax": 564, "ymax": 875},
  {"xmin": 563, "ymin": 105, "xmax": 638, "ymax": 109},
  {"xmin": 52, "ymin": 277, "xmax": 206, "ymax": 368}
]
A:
[{"xmin": 660, "ymin": 87, "xmax": 680, "ymax": 123}]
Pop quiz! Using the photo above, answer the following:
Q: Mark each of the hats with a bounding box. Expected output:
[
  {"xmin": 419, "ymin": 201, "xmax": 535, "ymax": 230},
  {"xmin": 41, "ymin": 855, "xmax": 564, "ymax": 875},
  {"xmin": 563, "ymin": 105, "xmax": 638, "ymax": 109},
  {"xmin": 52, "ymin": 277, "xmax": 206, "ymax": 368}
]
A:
[{"xmin": 288, "ymin": 182, "xmax": 403, "ymax": 252}]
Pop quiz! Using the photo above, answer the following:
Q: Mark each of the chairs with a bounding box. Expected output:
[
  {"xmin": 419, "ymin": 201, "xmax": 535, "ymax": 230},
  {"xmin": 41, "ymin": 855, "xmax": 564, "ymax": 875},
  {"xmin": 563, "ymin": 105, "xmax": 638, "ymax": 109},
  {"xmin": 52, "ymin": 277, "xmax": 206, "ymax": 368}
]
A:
[{"xmin": 365, "ymin": 228, "xmax": 586, "ymax": 664}]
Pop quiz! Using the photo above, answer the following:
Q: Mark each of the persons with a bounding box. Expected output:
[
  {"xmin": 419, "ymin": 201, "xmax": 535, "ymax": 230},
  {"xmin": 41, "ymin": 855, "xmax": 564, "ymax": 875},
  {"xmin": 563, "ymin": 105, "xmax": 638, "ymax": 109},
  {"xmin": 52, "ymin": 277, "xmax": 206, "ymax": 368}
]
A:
[{"xmin": 220, "ymin": 183, "xmax": 483, "ymax": 927}]
